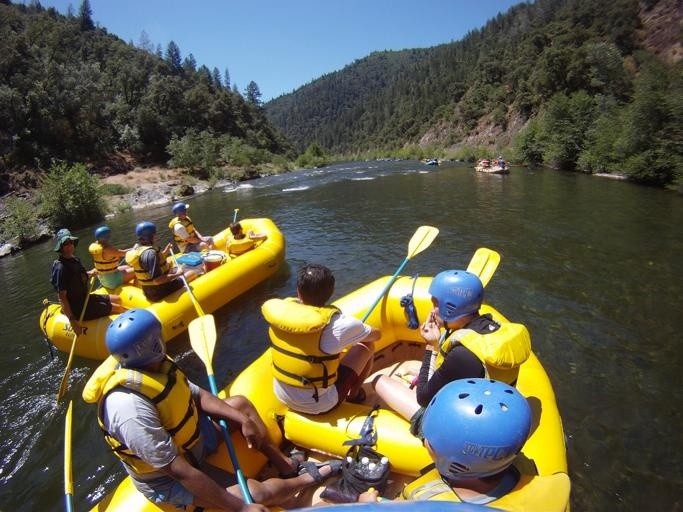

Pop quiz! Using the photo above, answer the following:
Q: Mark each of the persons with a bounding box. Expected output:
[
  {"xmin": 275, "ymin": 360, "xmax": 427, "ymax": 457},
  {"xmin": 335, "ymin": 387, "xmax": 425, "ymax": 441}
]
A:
[
  {"xmin": 372, "ymin": 269, "xmax": 531, "ymax": 445},
  {"xmin": 287, "ymin": 500, "xmax": 506, "ymax": 512},
  {"xmin": 89, "ymin": 227, "xmax": 138, "ymax": 289},
  {"xmin": 321, "ymin": 377, "xmax": 532, "ymax": 504},
  {"xmin": 82, "ymin": 309, "xmax": 346, "ymax": 512},
  {"xmin": 50, "ymin": 228, "xmax": 127, "ymax": 336},
  {"xmin": 169, "ymin": 202, "xmax": 214, "ymax": 254},
  {"xmin": 125, "ymin": 221, "xmax": 197, "ymax": 303},
  {"xmin": 227, "ymin": 222, "xmax": 267, "ymax": 257},
  {"xmin": 478, "ymin": 155, "xmax": 505, "ymax": 169},
  {"xmin": 261, "ymin": 264, "xmax": 382, "ymax": 416}
]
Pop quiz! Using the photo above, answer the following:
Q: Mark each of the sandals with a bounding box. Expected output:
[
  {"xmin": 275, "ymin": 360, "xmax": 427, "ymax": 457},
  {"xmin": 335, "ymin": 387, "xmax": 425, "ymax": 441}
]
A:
[
  {"xmin": 298, "ymin": 456, "xmax": 345, "ymax": 484},
  {"xmin": 278, "ymin": 447, "xmax": 307, "ymax": 479}
]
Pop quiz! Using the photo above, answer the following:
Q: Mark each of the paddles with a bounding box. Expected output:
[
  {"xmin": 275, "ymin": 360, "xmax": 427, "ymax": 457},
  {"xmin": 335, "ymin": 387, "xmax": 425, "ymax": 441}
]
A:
[
  {"xmin": 169, "ymin": 245, "xmax": 204, "ymax": 316},
  {"xmin": 63, "ymin": 400, "xmax": 73, "ymax": 510},
  {"xmin": 410, "ymin": 248, "xmax": 502, "ymax": 388},
  {"xmin": 188, "ymin": 315, "xmax": 254, "ymax": 504},
  {"xmin": 362, "ymin": 225, "xmax": 439, "ymax": 323},
  {"xmin": 57, "ymin": 275, "xmax": 95, "ymax": 403}
]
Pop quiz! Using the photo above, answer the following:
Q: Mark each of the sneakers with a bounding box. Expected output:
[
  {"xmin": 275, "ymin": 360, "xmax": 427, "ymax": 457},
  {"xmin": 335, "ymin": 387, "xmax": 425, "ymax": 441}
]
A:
[{"xmin": 346, "ymin": 388, "xmax": 366, "ymax": 403}]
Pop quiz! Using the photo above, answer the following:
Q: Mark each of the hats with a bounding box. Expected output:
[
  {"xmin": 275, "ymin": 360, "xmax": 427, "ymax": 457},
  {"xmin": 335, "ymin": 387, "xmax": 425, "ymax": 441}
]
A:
[{"xmin": 53, "ymin": 228, "xmax": 79, "ymax": 252}]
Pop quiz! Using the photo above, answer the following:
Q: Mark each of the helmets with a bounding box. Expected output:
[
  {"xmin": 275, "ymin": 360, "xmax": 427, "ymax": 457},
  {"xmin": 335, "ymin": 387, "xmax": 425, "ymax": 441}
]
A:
[
  {"xmin": 105, "ymin": 308, "xmax": 167, "ymax": 368},
  {"xmin": 422, "ymin": 378, "xmax": 533, "ymax": 481},
  {"xmin": 95, "ymin": 227, "xmax": 111, "ymax": 242},
  {"xmin": 136, "ymin": 222, "xmax": 156, "ymax": 240},
  {"xmin": 429, "ymin": 269, "xmax": 483, "ymax": 321},
  {"xmin": 172, "ymin": 203, "xmax": 185, "ymax": 215}
]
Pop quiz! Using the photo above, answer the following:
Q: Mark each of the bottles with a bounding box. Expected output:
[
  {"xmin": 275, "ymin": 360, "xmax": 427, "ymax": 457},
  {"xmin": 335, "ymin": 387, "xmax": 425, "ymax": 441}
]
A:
[{"xmin": 346, "ymin": 456, "xmax": 389, "ymax": 480}]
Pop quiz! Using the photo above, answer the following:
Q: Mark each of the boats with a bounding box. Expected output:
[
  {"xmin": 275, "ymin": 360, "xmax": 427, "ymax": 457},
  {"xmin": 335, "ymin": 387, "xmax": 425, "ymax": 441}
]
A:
[
  {"xmin": 474, "ymin": 166, "xmax": 511, "ymax": 175},
  {"xmin": 37, "ymin": 214, "xmax": 284, "ymax": 358},
  {"xmin": 86, "ymin": 272, "xmax": 574, "ymax": 512},
  {"xmin": 424, "ymin": 161, "xmax": 437, "ymax": 166}
]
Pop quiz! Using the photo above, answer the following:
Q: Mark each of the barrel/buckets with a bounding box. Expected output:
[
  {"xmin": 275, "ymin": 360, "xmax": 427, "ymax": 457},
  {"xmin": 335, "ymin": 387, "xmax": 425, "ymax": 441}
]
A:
[{"xmin": 201, "ymin": 250, "xmax": 227, "ymax": 271}]
